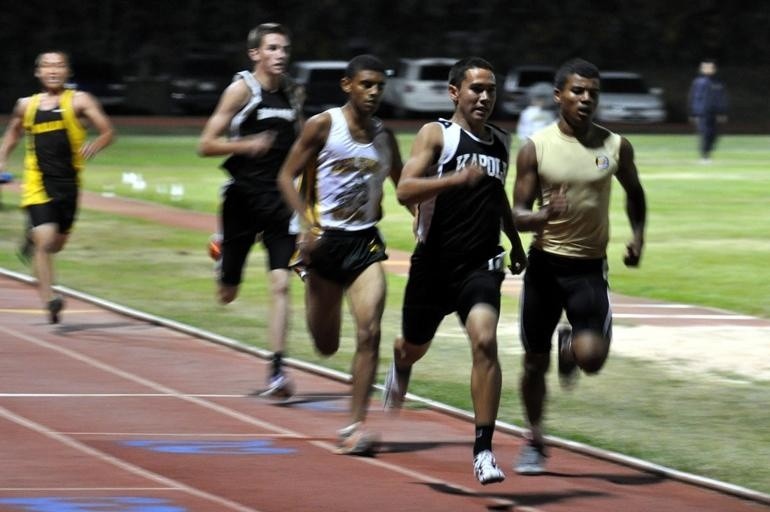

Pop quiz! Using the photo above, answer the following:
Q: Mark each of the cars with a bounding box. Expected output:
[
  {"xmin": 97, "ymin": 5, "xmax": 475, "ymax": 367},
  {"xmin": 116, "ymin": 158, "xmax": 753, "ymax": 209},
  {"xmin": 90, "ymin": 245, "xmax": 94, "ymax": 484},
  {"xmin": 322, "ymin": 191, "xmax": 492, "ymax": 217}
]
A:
[
  {"xmin": 590, "ymin": 72, "xmax": 669, "ymax": 128},
  {"xmin": 168, "ymin": 55, "xmax": 241, "ymax": 110},
  {"xmin": 291, "ymin": 58, "xmax": 348, "ymax": 117}
]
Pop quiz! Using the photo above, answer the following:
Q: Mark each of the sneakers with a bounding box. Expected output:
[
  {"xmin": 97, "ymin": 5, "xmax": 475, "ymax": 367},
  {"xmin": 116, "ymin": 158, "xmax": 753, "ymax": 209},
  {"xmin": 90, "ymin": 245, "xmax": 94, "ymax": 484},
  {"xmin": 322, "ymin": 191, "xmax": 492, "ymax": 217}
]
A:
[
  {"xmin": 339, "ymin": 418, "xmax": 383, "ymax": 452},
  {"xmin": 512, "ymin": 437, "xmax": 549, "ymax": 475},
  {"xmin": 262, "ymin": 373, "xmax": 293, "ymax": 394},
  {"xmin": 385, "ymin": 344, "xmax": 410, "ymax": 414},
  {"xmin": 45, "ymin": 296, "xmax": 63, "ymax": 324},
  {"xmin": 471, "ymin": 450, "xmax": 504, "ymax": 484}
]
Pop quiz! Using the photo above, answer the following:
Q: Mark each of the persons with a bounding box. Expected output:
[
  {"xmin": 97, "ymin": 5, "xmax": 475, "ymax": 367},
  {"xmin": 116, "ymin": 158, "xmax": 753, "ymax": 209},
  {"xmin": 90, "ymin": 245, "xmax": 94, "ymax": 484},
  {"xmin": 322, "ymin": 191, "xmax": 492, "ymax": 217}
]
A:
[
  {"xmin": 686, "ymin": 50, "xmax": 731, "ymax": 164},
  {"xmin": 276, "ymin": 51, "xmax": 415, "ymax": 458},
  {"xmin": 195, "ymin": 18, "xmax": 307, "ymax": 402},
  {"xmin": 516, "ymin": 92, "xmax": 556, "ymax": 148},
  {"xmin": 396, "ymin": 52, "xmax": 527, "ymax": 487},
  {"xmin": 0, "ymin": 47, "xmax": 117, "ymax": 328},
  {"xmin": 511, "ymin": 57, "xmax": 648, "ymax": 478}
]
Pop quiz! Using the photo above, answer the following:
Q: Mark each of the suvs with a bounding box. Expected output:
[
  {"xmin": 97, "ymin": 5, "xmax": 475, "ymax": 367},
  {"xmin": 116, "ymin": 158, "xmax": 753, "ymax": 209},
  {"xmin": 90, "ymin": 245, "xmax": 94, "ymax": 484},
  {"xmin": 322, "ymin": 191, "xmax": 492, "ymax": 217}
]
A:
[
  {"xmin": 384, "ymin": 58, "xmax": 457, "ymax": 118},
  {"xmin": 501, "ymin": 65, "xmax": 558, "ymax": 115}
]
